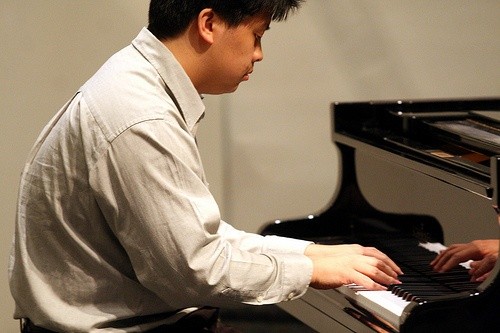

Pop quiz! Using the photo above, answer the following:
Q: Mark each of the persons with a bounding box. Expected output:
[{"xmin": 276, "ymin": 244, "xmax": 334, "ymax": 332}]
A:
[
  {"xmin": 430, "ymin": 239, "xmax": 500, "ymax": 282},
  {"xmin": 8, "ymin": 1, "xmax": 404, "ymax": 332}
]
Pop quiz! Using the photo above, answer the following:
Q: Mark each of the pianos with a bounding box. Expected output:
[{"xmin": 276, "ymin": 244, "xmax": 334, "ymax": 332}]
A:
[{"xmin": 257, "ymin": 94, "xmax": 500, "ymax": 333}]
